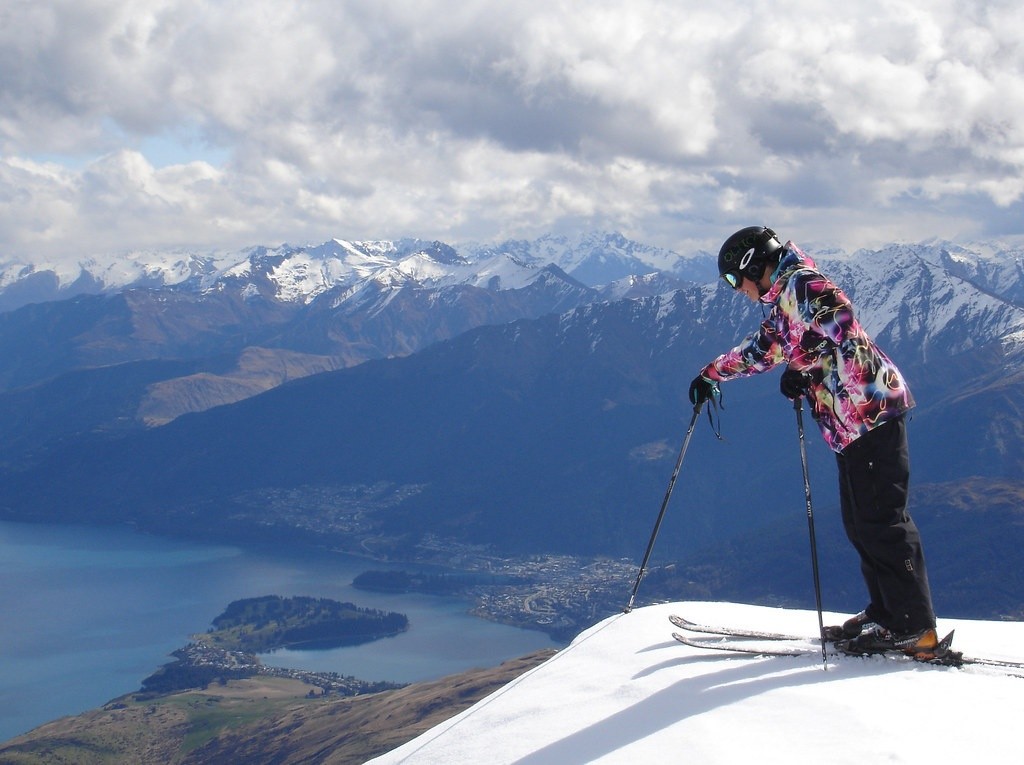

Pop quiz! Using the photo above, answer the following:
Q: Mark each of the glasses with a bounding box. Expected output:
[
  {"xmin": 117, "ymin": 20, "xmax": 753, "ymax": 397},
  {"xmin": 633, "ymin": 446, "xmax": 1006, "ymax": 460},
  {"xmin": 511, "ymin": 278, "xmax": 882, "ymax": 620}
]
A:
[{"xmin": 721, "ymin": 270, "xmax": 742, "ymax": 289}]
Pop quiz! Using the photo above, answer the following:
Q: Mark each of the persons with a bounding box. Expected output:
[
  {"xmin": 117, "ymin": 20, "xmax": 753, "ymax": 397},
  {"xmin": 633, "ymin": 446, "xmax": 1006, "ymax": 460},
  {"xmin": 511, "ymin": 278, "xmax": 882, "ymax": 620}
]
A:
[{"xmin": 687, "ymin": 226, "xmax": 941, "ymax": 659}]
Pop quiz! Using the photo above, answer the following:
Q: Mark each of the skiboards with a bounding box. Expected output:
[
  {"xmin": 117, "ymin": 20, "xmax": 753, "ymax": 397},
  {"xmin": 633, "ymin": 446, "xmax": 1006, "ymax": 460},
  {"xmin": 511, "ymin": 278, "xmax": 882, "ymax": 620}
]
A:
[{"xmin": 667, "ymin": 613, "xmax": 1023, "ymax": 670}]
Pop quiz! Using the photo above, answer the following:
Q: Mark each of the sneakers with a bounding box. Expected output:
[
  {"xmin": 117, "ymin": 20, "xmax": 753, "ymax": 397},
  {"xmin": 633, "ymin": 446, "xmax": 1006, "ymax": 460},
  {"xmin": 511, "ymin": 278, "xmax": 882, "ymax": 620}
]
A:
[
  {"xmin": 854, "ymin": 625, "xmax": 938, "ymax": 656},
  {"xmin": 843, "ymin": 609, "xmax": 881, "ymax": 635}
]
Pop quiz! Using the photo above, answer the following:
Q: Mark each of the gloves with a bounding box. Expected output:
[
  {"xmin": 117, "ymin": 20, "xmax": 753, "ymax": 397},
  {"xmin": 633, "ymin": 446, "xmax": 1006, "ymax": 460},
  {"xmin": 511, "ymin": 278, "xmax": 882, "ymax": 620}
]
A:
[
  {"xmin": 689, "ymin": 375, "xmax": 715, "ymax": 405},
  {"xmin": 780, "ymin": 367, "xmax": 812, "ymax": 399}
]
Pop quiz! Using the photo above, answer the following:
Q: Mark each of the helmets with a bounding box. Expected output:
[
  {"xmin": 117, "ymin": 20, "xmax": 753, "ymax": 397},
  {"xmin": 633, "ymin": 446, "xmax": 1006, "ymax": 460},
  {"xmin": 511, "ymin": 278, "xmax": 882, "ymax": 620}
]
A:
[{"xmin": 718, "ymin": 226, "xmax": 785, "ymax": 290}]
